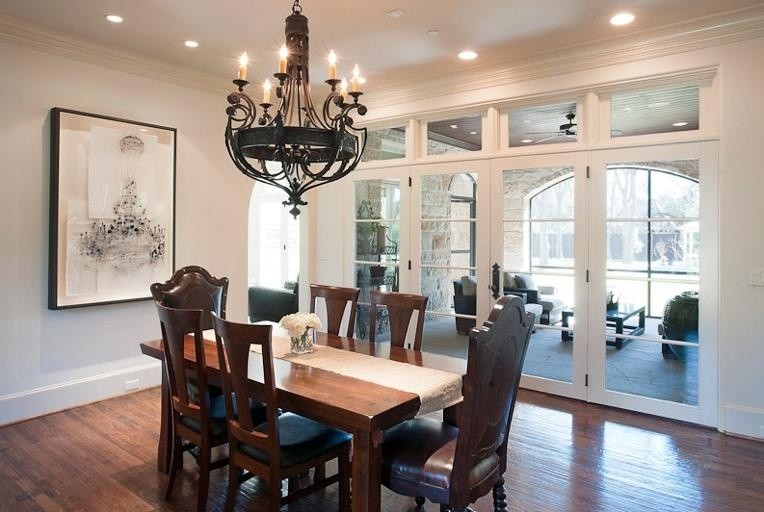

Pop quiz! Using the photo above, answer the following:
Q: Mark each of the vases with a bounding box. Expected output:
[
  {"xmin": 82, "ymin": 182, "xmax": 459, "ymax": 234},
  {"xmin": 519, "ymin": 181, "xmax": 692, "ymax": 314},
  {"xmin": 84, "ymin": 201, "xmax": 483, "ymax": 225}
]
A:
[{"xmin": 289, "ymin": 335, "xmax": 313, "ymax": 356}]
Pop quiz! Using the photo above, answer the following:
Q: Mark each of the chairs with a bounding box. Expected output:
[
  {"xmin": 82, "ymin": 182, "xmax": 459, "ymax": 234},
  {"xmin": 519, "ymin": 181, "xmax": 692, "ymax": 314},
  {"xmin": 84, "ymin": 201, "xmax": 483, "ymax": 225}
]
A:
[
  {"xmin": 369, "ymin": 288, "xmax": 430, "ymax": 351},
  {"xmin": 310, "ymin": 283, "xmax": 360, "ymax": 338},
  {"xmin": 154, "ymin": 301, "xmax": 268, "ymax": 512},
  {"xmin": 211, "ymin": 312, "xmax": 350, "ymax": 511},
  {"xmin": 380, "ymin": 294, "xmax": 534, "ymax": 512},
  {"xmin": 454, "ymin": 276, "xmax": 553, "ymax": 335},
  {"xmin": 150, "ymin": 266, "xmax": 228, "ymax": 473}
]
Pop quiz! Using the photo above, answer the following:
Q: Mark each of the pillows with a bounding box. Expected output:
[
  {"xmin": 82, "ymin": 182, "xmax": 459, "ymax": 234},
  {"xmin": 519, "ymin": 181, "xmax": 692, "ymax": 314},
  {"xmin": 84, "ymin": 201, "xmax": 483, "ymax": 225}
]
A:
[{"xmin": 515, "ymin": 275, "xmax": 542, "ymax": 299}]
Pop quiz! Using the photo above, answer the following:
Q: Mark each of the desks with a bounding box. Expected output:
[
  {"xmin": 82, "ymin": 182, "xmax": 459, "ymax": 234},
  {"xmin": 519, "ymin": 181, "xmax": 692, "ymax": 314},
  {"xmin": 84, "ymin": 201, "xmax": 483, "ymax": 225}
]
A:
[{"xmin": 140, "ymin": 325, "xmax": 467, "ymax": 512}]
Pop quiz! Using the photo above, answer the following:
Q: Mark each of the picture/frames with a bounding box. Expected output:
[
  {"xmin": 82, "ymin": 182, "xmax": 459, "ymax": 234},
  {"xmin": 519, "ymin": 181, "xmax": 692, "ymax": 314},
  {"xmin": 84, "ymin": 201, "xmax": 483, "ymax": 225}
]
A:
[{"xmin": 47, "ymin": 105, "xmax": 177, "ymax": 310}]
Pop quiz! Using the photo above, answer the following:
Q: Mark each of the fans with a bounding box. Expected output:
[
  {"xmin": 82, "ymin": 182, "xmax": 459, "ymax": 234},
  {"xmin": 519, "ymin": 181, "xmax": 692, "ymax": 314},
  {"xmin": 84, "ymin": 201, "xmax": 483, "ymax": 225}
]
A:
[{"xmin": 524, "ymin": 111, "xmax": 623, "ymax": 145}]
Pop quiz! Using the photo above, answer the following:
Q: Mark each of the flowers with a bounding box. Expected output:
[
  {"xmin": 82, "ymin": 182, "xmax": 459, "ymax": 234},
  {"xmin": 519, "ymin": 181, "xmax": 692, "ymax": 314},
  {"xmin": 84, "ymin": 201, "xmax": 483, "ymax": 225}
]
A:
[{"xmin": 279, "ymin": 311, "xmax": 322, "ymax": 349}]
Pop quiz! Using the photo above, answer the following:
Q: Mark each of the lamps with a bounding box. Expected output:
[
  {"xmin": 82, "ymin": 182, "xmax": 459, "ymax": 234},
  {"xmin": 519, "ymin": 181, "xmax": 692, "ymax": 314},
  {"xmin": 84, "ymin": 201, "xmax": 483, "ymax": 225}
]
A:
[{"xmin": 225, "ymin": 0, "xmax": 369, "ymax": 219}]
